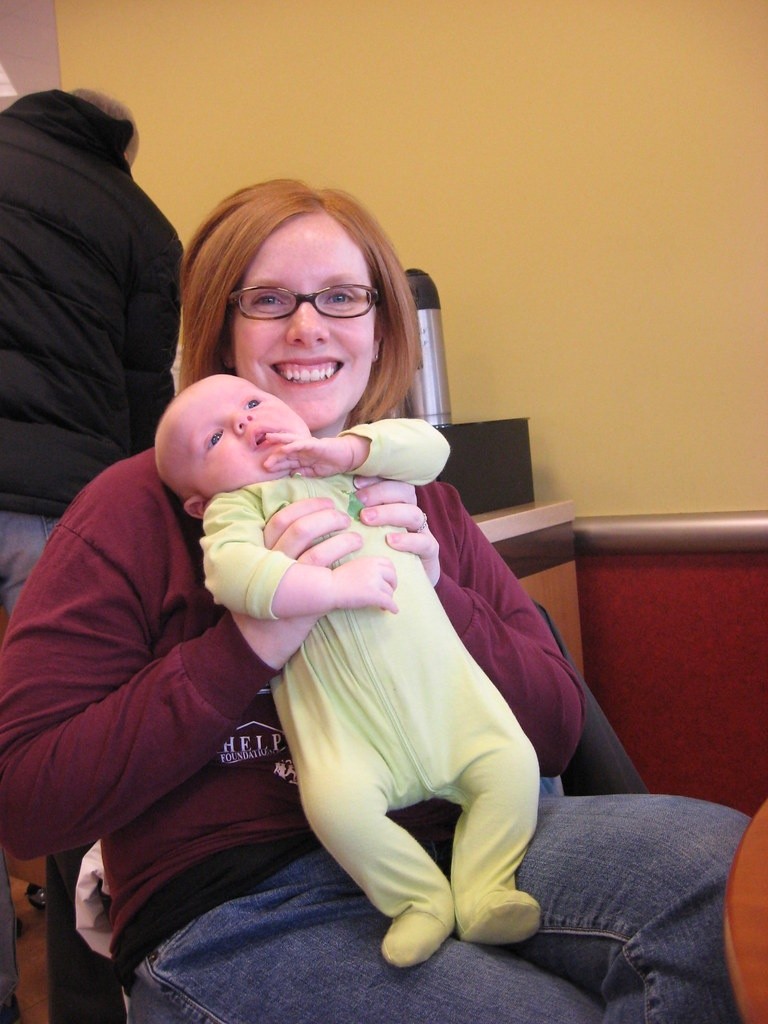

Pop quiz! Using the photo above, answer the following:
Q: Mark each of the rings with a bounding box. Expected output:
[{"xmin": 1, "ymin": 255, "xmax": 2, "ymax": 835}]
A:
[{"xmin": 415, "ymin": 512, "xmax": 427, "ymax": 533}]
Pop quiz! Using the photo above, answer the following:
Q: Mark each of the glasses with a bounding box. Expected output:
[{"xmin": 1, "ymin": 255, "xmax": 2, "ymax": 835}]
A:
[{"xmin": 228, "ymin": 284, "xmax": 378, "ymax": 321}]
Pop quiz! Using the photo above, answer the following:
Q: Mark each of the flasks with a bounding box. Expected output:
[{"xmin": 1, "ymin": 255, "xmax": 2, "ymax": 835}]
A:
[{"xmin": 401, "ymin": 268, "xmax": 452, "ymax": 429}]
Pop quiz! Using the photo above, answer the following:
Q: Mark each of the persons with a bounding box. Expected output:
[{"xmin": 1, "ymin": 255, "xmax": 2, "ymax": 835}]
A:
[
  {"xmin": 154, "ymin": 373, "xmax": 541, "ymax": 969},
  {"xmin": 0, "ymin": 90, "xmax": 185, "ymax": 625},
  {"xmin": 0, "ymin": 178, "xmax": 751, "ymax": 1024}
]
